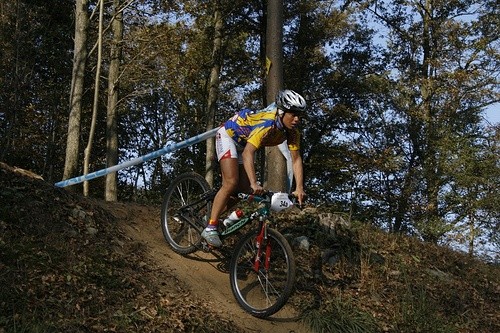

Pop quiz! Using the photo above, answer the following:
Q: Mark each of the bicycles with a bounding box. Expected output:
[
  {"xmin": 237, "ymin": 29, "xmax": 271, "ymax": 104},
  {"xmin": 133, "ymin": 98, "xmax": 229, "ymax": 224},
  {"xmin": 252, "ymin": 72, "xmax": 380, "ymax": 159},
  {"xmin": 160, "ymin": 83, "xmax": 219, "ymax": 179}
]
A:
[{"xmin": 160, "ymin": 157, "xmax": 306, "ymax": 319}]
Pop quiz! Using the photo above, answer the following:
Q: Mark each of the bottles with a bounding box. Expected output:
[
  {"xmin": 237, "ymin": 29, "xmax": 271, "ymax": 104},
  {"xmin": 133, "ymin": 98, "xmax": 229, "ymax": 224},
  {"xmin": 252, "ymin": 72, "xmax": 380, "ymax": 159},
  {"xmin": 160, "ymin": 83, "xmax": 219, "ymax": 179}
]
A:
[{"xmin": 223, "ymin": 210, "xmax": 243, "ymax": 227}]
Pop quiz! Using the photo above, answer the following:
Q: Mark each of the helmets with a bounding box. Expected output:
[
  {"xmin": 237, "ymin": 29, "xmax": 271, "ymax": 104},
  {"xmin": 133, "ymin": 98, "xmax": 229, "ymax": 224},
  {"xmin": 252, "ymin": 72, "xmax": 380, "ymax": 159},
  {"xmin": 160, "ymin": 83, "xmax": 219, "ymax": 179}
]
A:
[{"xmin": 274, "ymin": 89, "xmax": 306, "ymax": 113}]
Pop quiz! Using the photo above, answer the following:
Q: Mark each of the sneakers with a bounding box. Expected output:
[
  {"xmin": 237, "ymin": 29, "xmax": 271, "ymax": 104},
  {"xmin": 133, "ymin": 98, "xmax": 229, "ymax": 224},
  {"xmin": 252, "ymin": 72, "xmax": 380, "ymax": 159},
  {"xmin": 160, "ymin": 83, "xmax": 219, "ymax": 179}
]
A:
[
  {"xmin": 222, "ymin": 217, "xmax": 237, "ymax": 228},
  {"xmin": 200, "ymin": 228, "xmax": 222, "ymax": 247}
]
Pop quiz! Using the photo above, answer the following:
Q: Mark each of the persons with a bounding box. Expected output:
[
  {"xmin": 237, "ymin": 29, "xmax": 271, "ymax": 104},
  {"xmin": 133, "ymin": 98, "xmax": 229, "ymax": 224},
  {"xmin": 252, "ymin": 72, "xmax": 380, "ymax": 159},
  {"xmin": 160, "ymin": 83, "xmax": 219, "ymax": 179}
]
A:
[{"xmin": 201, "ymin": 89, "xmax": 307, "ymax": 247}]
